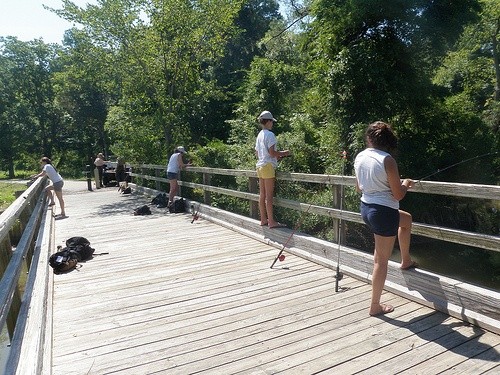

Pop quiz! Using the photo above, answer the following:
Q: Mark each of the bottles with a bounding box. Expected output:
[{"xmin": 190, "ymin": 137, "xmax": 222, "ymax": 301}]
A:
[
  {"xmin": 62, "ymin": 240, "xmax": 66, "ymax": 249},
  {"xmin": 56, "ymin": 245, "xmax": 62, "ymax": 252},
  {"xmin": 162, "ymin": 200, "xmax": 173, "ymax": 215}
]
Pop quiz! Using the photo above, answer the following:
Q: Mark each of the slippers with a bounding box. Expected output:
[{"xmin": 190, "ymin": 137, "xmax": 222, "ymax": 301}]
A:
[
  {"xmin": 370, "ymin": 303, "xmax": 395, "ymax": 316},
  {"xmin": 268, "ymin": 221, "xmax": 288, "ymax": 229},
  {"xmin": 260, "ymin": 218, "xmax": 269, "ymax": 226},
  {"xmin": 400, "ymin": 260, "xmax": 418, "ymax": 271}
]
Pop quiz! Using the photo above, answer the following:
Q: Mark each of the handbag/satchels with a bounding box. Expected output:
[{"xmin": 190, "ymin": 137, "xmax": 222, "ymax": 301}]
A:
[
  {"xmin": 48, "ymin": 250, "xmax": 78, "ymax": 275},
  {"xmin": 64, "ymin": 236, "xmax": 95, "ymax": 262}
]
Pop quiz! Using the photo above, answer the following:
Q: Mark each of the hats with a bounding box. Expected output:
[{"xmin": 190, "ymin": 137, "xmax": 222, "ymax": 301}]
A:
[
  {"xmin": 95, "ymin": 153, "xmax": 105, "ymax": 160},
  {"xmin": 177, "ymin": 145, "xmax": 187, "ymax": 154},
  {"xmin": 258, "ymin": 111, "xmax": 278, "ymax": 123}
]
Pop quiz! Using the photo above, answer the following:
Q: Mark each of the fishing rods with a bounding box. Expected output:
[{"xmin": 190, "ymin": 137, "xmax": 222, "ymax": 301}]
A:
[
  {"xmin": 334, "ymin": 150, "xmax": 347, "ymax": 293},
  {"xmin": 270, "ymin": 171, "xmax": 332, "ymax": 268},
  {"xmin": 190, "ymin": 191, "xmax": 207, "ymax": 223},
  {"xmin": 407, "ymin": 151, "xmax": 499, "ymax": 187}
]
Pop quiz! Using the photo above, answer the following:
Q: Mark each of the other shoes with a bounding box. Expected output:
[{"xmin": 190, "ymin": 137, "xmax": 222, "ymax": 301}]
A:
[
  {"xmin": 59, "ymin": 213, "xmax": 66, "ymax": 219},
  {"xmin": 168, "ymin": 201, "xmax": 175, "ymax": 210},
  {"xmin": 48, "ymin": 201, "xmax": 55, "ymax": 207}
]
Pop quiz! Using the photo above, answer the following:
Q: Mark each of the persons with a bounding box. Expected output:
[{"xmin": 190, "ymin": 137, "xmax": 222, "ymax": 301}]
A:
[
  {"xmin": 166, "ymin": 145, "xmax": 194, "ymax": 207},
  {"xmin": 33, "ymin": 156, "xmax": 66, "ymax": 216},
  {"xmin": 353, "ymin": 120, "xmax": 421, "ymax": 316},
  {"xmin": 255, "ymin": 110, "xmax": 292, "ymax": 227},
  {"xmin": 115, "ymin": 156, "xmax": 125, "ymax": 187},
  {"xmin": 93, "ymin": 152, "xmax": 104, "ymax": 189}
]
